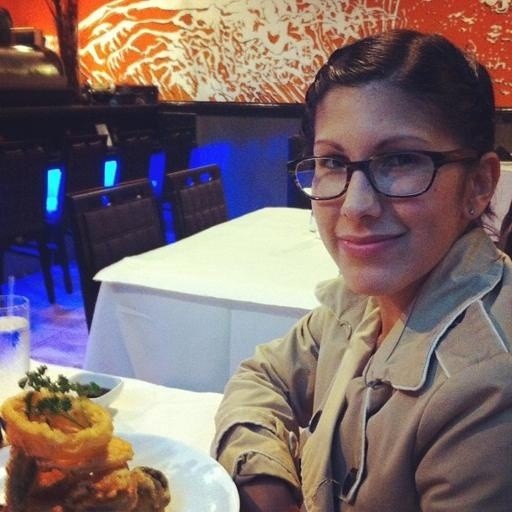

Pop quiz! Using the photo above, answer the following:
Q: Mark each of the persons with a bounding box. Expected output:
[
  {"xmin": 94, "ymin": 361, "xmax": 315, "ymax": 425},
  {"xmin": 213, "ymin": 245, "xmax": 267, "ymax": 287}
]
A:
[{"xmin": 208, "ymin": 26, "xmax": 511, "ymax": 510}]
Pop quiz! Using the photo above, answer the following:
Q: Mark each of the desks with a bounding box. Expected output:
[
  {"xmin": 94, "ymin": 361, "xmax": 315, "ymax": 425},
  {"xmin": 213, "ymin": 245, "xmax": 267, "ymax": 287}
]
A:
[
  {"xmin": 82, "ymin": 206, "xmax": 340, "ymax": 393},
  {"xmin": 29, "ymin": 360, "xmax": 224, "ymax": 457}
]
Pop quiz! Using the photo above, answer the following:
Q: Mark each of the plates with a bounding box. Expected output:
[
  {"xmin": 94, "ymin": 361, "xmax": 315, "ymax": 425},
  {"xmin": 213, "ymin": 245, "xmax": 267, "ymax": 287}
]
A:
[{"xmin": 0, "ymin": 435, "xmax": 241, "ymax": 512}]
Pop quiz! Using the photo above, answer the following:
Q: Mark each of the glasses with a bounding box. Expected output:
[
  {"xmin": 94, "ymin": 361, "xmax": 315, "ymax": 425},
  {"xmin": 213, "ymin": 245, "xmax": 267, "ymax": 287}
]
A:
[{"xmin": 293, "ymin": 148, "xmax": 480, "ymax": 198}]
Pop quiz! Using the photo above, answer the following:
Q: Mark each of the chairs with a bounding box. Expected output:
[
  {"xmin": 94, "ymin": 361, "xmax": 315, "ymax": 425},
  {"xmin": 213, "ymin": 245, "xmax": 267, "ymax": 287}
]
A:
[
  {"xmin": 63, "ymin": 177, "xmax": 168, "ymax": 330},
  {"xmin": 1, "ymin": 137, "xmax": 59, "ymax": 306},
  {"xmin": 150, "ymin": 110, "xmax": 198, "ymax": 207},
  {"xmin": 50, "ymin": 134, "xmax": 110, "ymax": 296},
  {"xmin": 163, "ymin": 162, "xmax": 227, "ymax": 242},
  {"xmin": 113, "ymin": 128, "xmax": 156, "ymax": 183}
]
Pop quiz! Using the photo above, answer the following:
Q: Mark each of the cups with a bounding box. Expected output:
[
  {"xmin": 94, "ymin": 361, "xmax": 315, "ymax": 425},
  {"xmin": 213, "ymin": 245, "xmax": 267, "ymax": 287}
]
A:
[{"xmin": 0, "ymin": 295, "xmax": 31, "ymax": 403}]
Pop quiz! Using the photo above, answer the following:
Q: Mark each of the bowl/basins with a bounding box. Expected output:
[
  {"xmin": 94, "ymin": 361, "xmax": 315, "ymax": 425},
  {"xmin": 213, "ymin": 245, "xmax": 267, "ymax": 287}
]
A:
[{"xmin": 68, "ymin": 372, "xmax": 124, "ymax": 409}]
